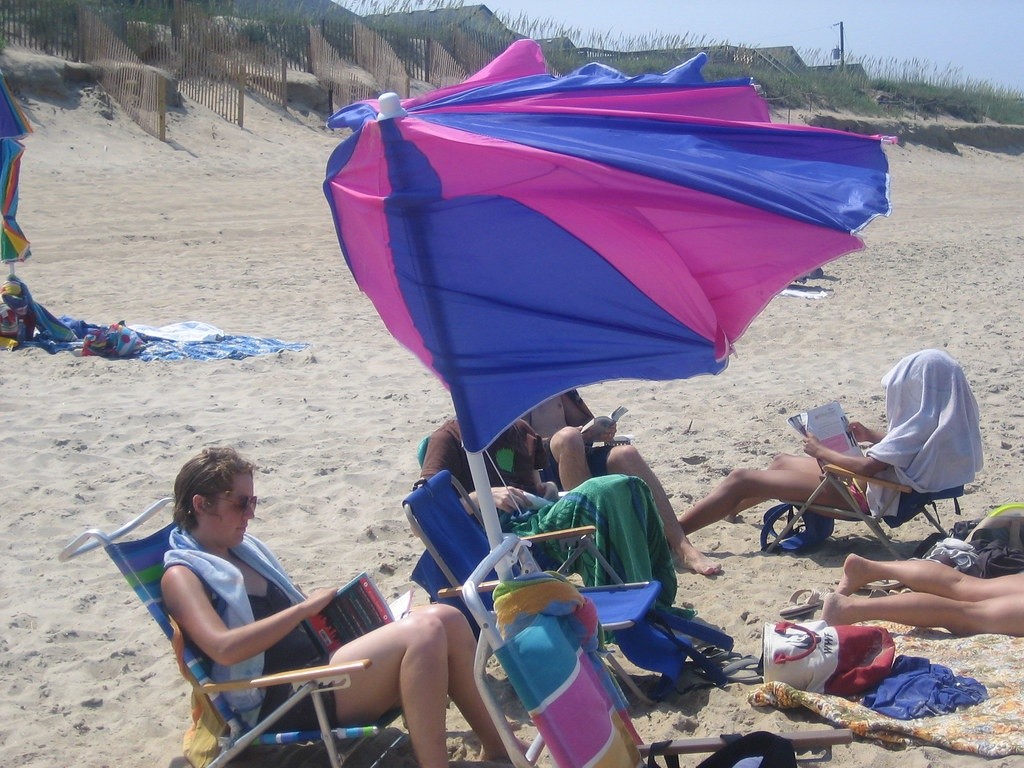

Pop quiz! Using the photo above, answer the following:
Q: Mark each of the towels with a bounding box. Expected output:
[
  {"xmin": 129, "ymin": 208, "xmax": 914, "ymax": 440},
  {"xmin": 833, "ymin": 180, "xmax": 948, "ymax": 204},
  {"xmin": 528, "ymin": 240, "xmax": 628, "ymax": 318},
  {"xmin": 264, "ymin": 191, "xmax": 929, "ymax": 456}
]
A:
[
  {"xmin": 507, "ymin": 475, "xmax": 699, "ymax": 645},
  {"xmin": 858, "ymin": 654, "xmax": 989, "ymax": 720},
  {"xmin": 865, "ymin": 348, "xmax": 984, "ymax": 516},
  {"xmin": 21, "ymin": 313, "xmax": 313, "ymax": 364},
  {"xmin": 164, "ymin": 527, "xmax": 311, "ymax": 728}
]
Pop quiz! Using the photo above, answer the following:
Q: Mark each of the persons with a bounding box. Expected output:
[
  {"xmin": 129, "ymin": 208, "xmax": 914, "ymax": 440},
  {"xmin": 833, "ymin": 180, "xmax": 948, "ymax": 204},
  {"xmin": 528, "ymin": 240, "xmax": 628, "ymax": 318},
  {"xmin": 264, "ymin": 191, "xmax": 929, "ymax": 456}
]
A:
[
  {"xmin": 821, "ymin": 553, "xmax": 1024, "ymax": 637},
  {"xmin": 160, "ymin": 446, "xmax": 536, "ymax": 768},
  {"xmin": 419, "ymin": 388, "xmax": 721, "ymax": 642},
  {"xmin": 666, "ymin": 350, "xmax": 981, "ymax": 545}
]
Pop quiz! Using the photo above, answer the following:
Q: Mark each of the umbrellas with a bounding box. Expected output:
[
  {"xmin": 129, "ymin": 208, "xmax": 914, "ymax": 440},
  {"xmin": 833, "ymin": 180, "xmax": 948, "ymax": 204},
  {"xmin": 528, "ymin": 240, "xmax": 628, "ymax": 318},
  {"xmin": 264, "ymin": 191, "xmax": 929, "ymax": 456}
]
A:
[
  {"xmin": 323, "ymin": 39, "xmax": 892, "ymax": 583},
  {"xmin": 0, "ymin": 66, "xmax": 35, "ymax": 277}
]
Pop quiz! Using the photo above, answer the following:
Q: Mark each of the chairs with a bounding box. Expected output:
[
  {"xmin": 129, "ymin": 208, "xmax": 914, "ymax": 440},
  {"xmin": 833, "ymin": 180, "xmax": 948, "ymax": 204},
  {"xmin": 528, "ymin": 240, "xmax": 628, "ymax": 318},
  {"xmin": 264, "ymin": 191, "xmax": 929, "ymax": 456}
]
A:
[
  {"xmin": 403, "ymin": 469, "xmax": 731, "ymax": 706},
  {"xmin": 759, "ymin": 427, "xmax": 970, "ymax": 561},
  {"xmin": 57, "ymin": 498, "xmax": 403, "ymax": 768},
  {"xmin": 462, "ymin": 534, "xmax": 853, "ymax": 768}
]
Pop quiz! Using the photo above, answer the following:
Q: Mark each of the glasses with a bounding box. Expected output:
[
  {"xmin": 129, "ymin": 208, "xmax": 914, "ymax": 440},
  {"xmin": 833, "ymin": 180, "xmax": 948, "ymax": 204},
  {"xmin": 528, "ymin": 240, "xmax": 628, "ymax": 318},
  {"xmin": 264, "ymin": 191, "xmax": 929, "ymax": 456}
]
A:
[{"xmin": 210, "ymin": 495, "xmax": 257, "ymax": 513}]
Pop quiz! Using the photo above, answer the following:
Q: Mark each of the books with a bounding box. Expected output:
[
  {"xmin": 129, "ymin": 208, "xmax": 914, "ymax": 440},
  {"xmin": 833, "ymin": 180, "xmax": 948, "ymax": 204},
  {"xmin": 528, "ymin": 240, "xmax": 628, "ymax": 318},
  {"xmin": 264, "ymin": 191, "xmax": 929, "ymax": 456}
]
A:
[
  {"xmin": 786, "ymin": 399, "xmax": 866, "ymax": 473},
  {"xmin": 308, "ymin": 571, "xmax": 414, "ymax": 653},
  {"xmin": 580, "ymin": 405, "xmax": 629, "ymax": 434}
]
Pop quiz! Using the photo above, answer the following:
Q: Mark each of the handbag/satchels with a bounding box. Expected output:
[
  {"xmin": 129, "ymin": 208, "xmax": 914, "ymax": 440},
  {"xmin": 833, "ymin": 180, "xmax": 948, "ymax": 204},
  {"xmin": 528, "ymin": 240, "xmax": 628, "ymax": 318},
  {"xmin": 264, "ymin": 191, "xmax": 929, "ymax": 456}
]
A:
[{"xmin": 721, "ymin": 620, "xmax": 896, "ymax": 699}]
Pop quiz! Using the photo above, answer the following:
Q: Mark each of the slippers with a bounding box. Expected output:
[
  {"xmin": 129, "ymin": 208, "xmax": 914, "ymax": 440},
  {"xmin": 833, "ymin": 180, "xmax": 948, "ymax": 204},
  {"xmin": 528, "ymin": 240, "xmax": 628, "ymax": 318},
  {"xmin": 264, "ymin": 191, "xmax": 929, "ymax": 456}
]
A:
[
  {"xmin": 857, "ymin": 579, "xmax": 905, "ymax": 591},
  {"xmin": 779, "ymin": 588, "xmax": 827, "ymax": 620}
]
